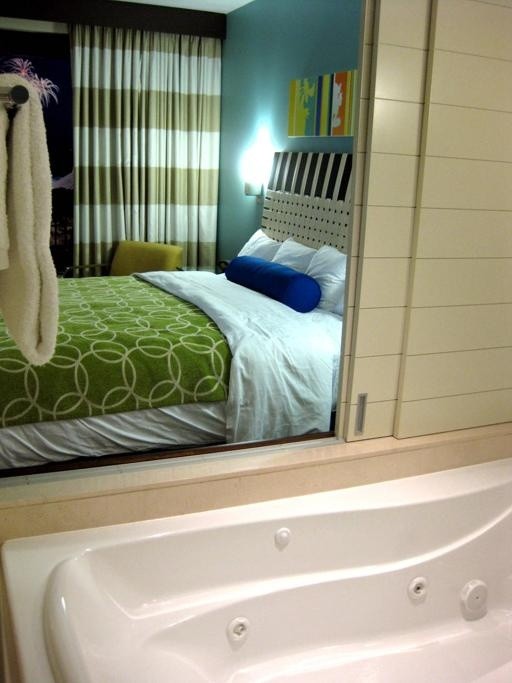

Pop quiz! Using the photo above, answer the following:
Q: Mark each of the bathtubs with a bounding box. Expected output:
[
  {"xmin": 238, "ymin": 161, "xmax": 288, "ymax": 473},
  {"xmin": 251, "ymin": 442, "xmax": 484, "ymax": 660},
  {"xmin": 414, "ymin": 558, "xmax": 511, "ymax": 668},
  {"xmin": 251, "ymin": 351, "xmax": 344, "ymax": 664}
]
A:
[{"xmin": 2, "ymin": 457, "xmax": 512, "ymax": 683}]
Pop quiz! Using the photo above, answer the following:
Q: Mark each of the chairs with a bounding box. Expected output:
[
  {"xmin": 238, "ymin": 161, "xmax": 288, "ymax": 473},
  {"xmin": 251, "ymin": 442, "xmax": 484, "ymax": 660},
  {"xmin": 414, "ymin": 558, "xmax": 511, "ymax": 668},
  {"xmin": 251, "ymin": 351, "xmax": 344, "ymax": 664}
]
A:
[{"xmin": 61, "ymin": 239, "xmax": 185, "ymax": 280}]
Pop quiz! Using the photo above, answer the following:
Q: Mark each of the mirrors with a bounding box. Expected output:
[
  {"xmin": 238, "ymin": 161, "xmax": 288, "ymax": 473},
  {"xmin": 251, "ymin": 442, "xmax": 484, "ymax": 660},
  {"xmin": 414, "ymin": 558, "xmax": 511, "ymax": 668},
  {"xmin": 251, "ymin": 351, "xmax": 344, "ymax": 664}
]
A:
[{"xmin": 0, "ymin": 1, "xmax": 364, "ymax": 489}]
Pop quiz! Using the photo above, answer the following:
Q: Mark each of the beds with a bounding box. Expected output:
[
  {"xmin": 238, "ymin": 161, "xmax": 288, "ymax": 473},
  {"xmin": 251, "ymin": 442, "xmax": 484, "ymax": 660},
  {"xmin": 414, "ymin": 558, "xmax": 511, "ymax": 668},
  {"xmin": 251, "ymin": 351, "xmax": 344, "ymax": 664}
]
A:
[{"xmin": 1, "ymin": 151, "xmax": 355, "ymax": 475}]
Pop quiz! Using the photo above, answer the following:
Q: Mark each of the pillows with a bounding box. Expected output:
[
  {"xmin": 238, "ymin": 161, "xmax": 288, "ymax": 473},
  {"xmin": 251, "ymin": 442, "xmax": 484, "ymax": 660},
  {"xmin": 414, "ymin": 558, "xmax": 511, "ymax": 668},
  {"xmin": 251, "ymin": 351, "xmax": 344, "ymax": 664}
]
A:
[
  {"xmin": 270, "ymin": 236, "xmax": 318, "ymax": 274},
  {"xmin": 237, "ymin": 228, "xmax": 282, "ymax": 262},
  {"xmin": 304, "ymin": 244, "xmax": 348, "ymax": 316},
  {"xmin": 225, "ymin": 255, "xmax": 321, "ymax": 313}
]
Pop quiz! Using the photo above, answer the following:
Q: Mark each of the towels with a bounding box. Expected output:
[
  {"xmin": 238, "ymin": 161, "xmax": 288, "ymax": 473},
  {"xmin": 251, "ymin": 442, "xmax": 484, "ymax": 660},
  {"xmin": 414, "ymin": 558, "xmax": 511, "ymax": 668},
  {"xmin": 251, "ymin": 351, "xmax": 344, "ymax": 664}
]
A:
[{"xmin": 0, "ymin": 71, "xmax": 60, "ymax": 367}]
